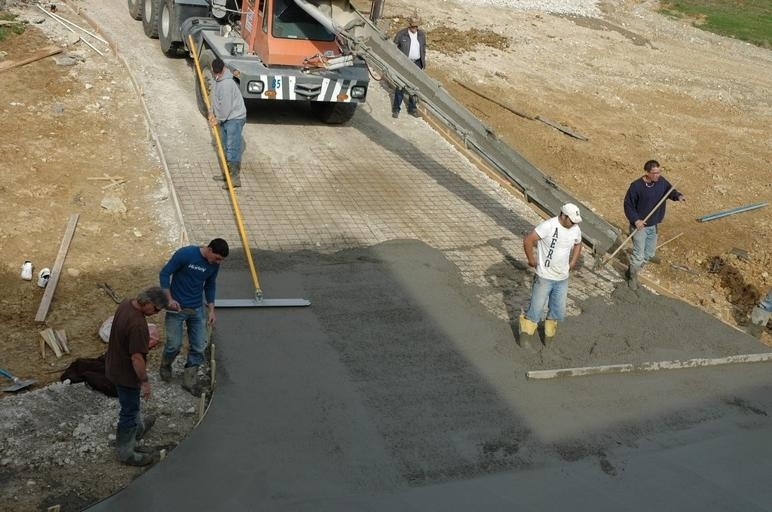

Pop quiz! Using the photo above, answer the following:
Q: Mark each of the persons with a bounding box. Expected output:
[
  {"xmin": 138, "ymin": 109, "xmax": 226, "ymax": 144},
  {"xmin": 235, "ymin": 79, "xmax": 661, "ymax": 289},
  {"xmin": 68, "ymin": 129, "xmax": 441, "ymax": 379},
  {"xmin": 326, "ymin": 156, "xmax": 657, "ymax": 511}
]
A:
[
  {"xmin": 519, "ymin": 203, "xmax": 582, "ymax": 348},
  {"xmin": 624, "ymin": 159, "xmax": 686, "ymax": 290},
  {"xmin": 393, "ymin": 17, "xmax": 427, "ymax": 118},
  {"xmin": 208, "ymin": 58, "xmax": 248, "ymax": 189},
  {"xmin": 105, "ymin": 285, "xmax": 168, "ymax": 465},
  {"xmin": 160, "ymin": 238, "xmax": 230, "ymax": 395}
]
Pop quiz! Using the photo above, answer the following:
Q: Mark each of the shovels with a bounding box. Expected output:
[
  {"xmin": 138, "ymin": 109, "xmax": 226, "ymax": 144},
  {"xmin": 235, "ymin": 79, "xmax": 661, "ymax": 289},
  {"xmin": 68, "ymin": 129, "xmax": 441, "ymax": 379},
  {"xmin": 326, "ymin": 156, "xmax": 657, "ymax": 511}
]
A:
[
  {"xmin": 0, "ymin": 368, "xmax": 38, "ymax": 391},
  {"xmin": 592, "ymin": 178, "xmax": 681, "ymax": 271}
]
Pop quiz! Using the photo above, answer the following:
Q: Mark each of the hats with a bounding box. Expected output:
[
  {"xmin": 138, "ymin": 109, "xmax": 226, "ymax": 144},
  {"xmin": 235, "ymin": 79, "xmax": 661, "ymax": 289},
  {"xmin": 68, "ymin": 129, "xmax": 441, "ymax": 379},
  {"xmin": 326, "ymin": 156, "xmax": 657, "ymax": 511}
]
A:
[
  {"xmin": 409, "ymin": 14, "xmax": 420, "ymax": 26},
  {"xmin": 560, "ymin": 202, "xmax": 583, "ymax": 224},
  {"xmin": 211, "ymin": 59, "xmax": 224, "ymax": 72}
]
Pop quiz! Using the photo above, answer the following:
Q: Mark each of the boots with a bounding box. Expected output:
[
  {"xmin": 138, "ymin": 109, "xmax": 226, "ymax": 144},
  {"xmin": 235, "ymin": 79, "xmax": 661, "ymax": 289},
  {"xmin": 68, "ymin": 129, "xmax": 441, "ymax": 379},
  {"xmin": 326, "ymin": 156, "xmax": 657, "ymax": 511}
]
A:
[
  {"xmin": 222, "ymin": 160, "xmax": 242, "ymax": 190},
  {"xmin": 213, "ymin": 172, "xmax": 227, "ymax": 181},
  {"xmin": 159, "ymin": 349, "xmax": 181, "ymax": 382},
  {"xmin": 181, "ymin": 363, "xmax": 202, "ymax": 397}
]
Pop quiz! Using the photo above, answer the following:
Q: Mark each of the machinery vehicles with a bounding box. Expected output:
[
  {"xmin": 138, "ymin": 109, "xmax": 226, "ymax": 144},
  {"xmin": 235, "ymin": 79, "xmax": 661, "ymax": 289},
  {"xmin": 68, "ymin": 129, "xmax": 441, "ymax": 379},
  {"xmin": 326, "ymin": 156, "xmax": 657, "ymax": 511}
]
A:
[{"xmin": 128, "ymin": 0, "xmax": 626, "ymax": 258}]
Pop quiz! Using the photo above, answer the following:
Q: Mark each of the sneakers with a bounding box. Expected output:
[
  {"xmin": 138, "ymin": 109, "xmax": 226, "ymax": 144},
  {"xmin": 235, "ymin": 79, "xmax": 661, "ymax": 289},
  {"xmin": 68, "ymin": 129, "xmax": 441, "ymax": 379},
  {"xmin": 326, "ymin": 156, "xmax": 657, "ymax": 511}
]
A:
[
  {"xmin": 37, "ymin": 268, "xmax": 50, "ymax": 288},
  {"xmin": 135, "ymin": 414, "xmax": 156, "ymax": 442},
  {"xmin": 20, "ymin": 261, "xmax": 33, "ymax": 280},
  {"xmin": 123, "ymin": 452, "xmax": 153, "ymax": 467}
]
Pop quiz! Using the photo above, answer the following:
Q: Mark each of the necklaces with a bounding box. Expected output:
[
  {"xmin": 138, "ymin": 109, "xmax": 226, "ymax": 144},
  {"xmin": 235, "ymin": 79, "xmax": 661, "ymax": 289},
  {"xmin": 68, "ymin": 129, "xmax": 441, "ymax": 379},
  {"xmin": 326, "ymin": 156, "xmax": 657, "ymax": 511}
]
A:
[{"xmin": 645, "ymin": 176, "xmax": 655, "ymax": 188}]
